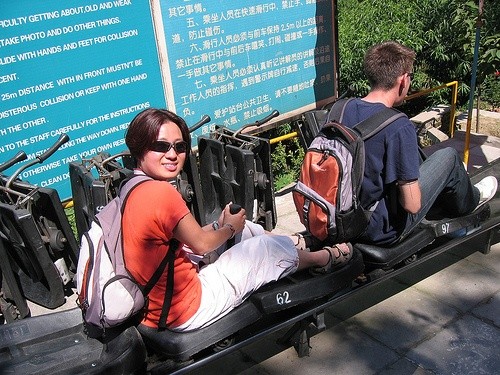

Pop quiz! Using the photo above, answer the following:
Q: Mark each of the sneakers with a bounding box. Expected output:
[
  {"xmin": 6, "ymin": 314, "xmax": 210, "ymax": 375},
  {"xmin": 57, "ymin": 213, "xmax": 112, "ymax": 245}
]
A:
[{"xmin": 471, "ymin": 176, "xmax": 498, "ymax": 214}]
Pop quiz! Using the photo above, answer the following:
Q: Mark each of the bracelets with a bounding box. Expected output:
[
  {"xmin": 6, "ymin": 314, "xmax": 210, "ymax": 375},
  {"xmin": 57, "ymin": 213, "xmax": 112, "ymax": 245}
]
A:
[
  {"xmin": 224, "ymin": 224, "xmax": 235, "ymax": 240},
  {"xmin": 212, "ymin": 221, "xmax": 219, "ymax": 231}
]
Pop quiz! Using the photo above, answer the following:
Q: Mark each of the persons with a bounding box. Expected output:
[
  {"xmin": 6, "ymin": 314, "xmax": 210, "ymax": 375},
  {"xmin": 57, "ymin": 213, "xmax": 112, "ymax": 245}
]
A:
[
  {"xmin": 120, "ymin": 107, "xmax": 354, "ymax": 333},
  {"xmin": 325, "ymin": 41, "xmax": 498, "ymax": 248}
]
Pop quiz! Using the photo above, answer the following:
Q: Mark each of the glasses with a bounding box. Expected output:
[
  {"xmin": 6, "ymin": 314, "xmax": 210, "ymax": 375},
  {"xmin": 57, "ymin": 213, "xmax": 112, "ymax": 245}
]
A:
[{"xmin": 144, "ymin": 141, "xmax": 188, "ymax": 153}]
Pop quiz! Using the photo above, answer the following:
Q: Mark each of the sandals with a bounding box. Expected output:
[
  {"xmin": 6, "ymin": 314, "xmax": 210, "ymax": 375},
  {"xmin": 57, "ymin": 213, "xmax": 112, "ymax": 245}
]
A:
[
  {"xmin": 309, "ymin": 242, "xmax": 353, "ymax": 275},
  {"xmin": 295, "ymin": 234, "xmax": 319, "ymax": 251}
]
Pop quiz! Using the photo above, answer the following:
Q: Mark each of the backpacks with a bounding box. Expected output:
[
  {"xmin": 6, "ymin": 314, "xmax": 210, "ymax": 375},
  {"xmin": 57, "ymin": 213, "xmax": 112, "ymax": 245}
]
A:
[
  {"xmin": 76, "ymin": 176, "xmax": 182, "ymax": 344},
  {"xmin": 291, "ymin": 98, "xmax": 408, "ymax": 247}
]
